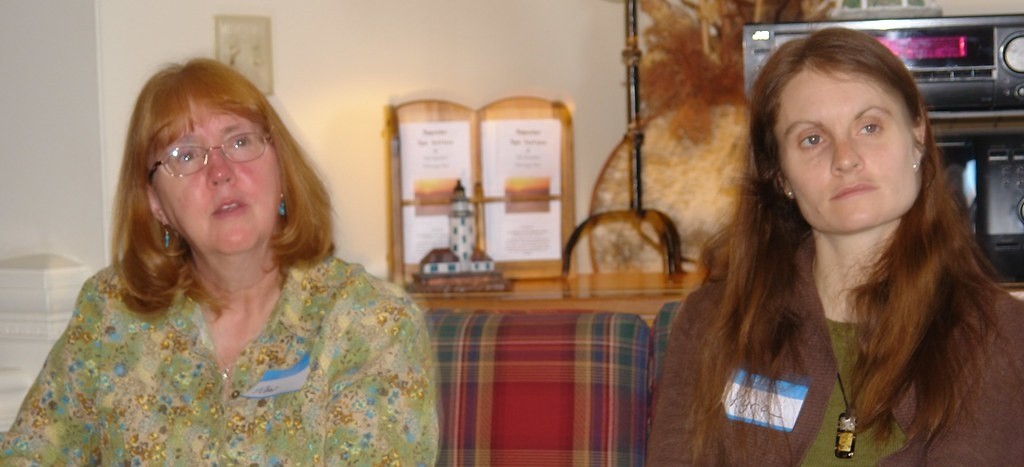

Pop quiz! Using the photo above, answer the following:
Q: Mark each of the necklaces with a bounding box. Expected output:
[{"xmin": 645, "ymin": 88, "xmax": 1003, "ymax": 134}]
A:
[
  {"xmin": 835, "ymin": 370, "xmax": 859, "ymax": 458},
  {"xmin": 214, "ymin": 341, "xmax": 231, "ymax": 380}
]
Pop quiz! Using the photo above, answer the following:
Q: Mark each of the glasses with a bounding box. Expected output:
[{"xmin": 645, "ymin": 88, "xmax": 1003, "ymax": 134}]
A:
[{"xmin": 146, "ymin": 132, "xmax": 273, "ymax": 186}]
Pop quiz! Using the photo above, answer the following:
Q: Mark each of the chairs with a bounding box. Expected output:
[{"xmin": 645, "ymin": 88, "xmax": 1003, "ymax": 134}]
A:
[{"xmin": 424, "ymin": 300, "xmax": 686, "ymax": 467}]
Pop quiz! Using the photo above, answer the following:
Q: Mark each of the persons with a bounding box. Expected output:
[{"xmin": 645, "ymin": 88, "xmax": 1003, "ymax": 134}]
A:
[
  {"xmin": 647, "ymin": 29, "xmax": 1024, "ymax": 467},
  {"xmin": 0, "ymin": 58, "xmax": 439, "ymax": 467}
]
